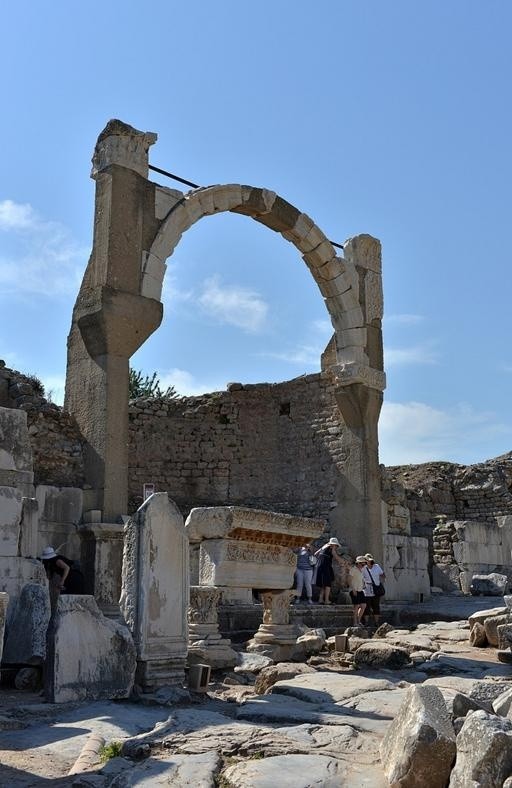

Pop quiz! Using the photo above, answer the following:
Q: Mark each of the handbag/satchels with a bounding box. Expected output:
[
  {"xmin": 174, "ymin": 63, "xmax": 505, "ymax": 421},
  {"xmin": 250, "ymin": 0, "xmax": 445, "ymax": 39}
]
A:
[
  {"xmin": 372, "ymin": 584, "xmax": 385, "ymax": 597},
  {"xmin": 308, "ymin": 555, "xmax": 317, "ymax": 566}
]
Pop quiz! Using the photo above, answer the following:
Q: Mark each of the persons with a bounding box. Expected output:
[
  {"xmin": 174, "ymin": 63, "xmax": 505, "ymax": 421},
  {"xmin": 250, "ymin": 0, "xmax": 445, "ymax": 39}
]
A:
[
  {"xmin": 37, "ymin": 544, "xmax": 87, "ymax": 596},
  {"xmin": 347, "ymin": 555, "xmax": 367, "ymax": 626},
  {"xmin": 292, "ymin": 542, "xmax": 316, "ymax": 605},
  {"xmin": 308, "ymin": 537, "xmax": 345, "ymax": 605},
  {"xmin": 361, "ymin": 552, "xmax": 386, "ymax": 628}
]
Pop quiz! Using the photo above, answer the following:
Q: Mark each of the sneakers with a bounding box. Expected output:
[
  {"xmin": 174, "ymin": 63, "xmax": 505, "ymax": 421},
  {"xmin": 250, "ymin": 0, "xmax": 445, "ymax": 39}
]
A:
[
  {"xmin": 353, "ymin": 625, "xmax": 360, "ymax": 627},
  {"xmin": 325, "ymin": 601, "xmax": 335, "ymax": 605},
  {"xmin": 359, "ymin": 623, "xmax": 365, "ymax": 627},
  {"xmin": 320, "ymin": 600, "xmax": 324, "ymax": 604},
  {"xmin": 308, "ymin": 600, "xmax": 312, "ymax": 604},
  {"xmin": 296, "ymin": 600, "xmax": 301, "ymax": 604}
]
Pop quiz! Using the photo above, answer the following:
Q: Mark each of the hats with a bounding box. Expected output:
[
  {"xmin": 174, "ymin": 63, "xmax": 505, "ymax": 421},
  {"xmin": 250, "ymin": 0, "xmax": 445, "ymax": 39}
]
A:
[
  {"xmin": 354, "ymin": 556, "xmax": 366, "ymax": 563},
  {"xmin": 364, "ymin": 553, "xmax": 375, "ymax": 561},
  {"xmin": 328, "ymin": 537, "xmax": 342, "ymax": 547}
]
